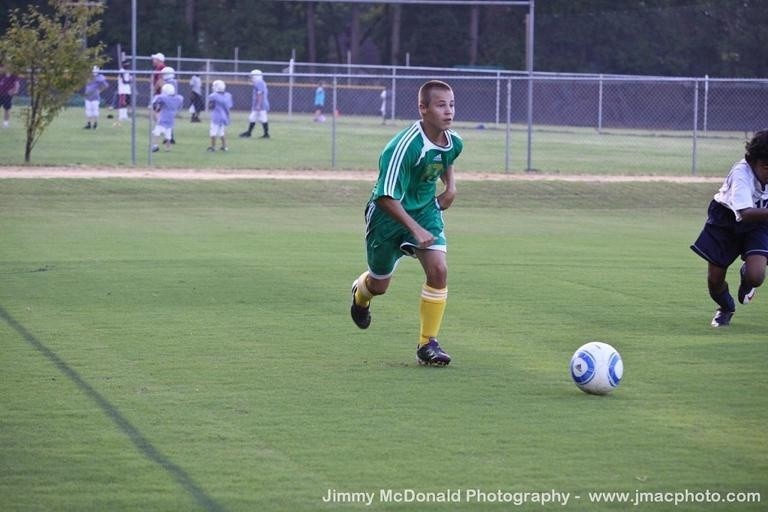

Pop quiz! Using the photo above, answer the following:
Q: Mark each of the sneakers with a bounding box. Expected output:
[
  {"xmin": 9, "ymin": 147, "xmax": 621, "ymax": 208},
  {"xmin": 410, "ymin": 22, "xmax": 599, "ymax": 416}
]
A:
[
  {"xmin": 351, "ymin": 279, "xmax": 371, "ymax": 329},
  {"xmin": 711, "ymin": 307, "xmax": 735, "ymax": 327},
  {"xmin": 416, "ymin": 338, "xmax": 451, "ymax": 367},
  {"xmin": 738, "ymin": 264, "xmax": 754, "ymax": 304}
]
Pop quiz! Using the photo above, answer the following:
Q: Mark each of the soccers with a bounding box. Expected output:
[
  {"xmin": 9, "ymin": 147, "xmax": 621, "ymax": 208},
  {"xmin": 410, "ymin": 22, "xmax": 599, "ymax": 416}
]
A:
[{"xmin": 571, "ymin": 342, "xmax": 624, "ymax": 394}]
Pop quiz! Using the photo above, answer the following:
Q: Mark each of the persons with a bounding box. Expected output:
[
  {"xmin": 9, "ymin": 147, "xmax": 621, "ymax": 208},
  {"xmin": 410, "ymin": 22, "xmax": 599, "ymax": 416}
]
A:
[
  {"xmin": 348, "ymin": 79, "xmax": 463, "ymax": 365},
  {"xmin": 0, "ymin": 52, "xmax": 389, "ymax": 154},
  {"xmin": 689, "ymin": 131, "xmax": 767, "ymax": 327}
]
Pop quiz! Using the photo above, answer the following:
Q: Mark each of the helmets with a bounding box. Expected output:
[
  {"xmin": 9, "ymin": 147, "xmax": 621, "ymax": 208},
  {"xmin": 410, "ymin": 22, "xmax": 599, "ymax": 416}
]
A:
[
  {"xmin": 152, "ymin": 54, "xmax": 176, "ymax": 95},
  {"xmin": 212, "ymin": 80, "xmax": 225, "ymax": 92},
  {"xmin": 251, "ymin": 70, "xmax": 262, "ymax": 81}
]
